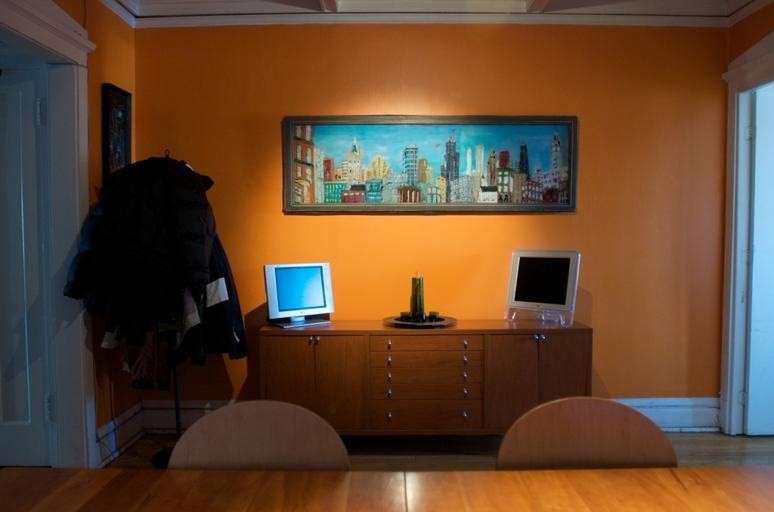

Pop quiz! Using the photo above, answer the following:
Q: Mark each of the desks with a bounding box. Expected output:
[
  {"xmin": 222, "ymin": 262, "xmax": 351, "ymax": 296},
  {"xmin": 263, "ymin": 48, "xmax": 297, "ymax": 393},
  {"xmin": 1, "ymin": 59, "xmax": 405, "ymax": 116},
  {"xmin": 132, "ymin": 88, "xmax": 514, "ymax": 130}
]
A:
[
  {"xmin": 0, "ymin": 466, "xmax": 406, "ymax": 511},
  {"xmin": 406, "ymin": 466, "xmax": 774, "ymax": 511}
]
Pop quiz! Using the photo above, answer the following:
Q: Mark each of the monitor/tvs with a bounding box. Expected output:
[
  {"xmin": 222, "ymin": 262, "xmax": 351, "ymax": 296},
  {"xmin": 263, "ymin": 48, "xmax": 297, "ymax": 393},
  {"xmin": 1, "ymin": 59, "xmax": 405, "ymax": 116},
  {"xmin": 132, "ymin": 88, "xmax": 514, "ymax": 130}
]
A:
[
  {"xmin": 263, "ymin": 262, "xmax": 335, "ymax": 329},
  {"xmin": 507, "ymin": 251, "xmax": 578, "ymax": 310}
]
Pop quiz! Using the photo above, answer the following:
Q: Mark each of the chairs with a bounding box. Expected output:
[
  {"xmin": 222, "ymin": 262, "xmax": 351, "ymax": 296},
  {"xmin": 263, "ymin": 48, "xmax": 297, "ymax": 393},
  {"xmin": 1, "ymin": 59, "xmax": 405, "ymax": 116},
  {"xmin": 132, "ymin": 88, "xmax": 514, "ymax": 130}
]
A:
[
  {"xmin": 164, "ymin": 398, "xmax": 354, "ymax": 466},
  {"xmin": 492, "ymin": 394, "xmax": 679, "ymax": 467}
]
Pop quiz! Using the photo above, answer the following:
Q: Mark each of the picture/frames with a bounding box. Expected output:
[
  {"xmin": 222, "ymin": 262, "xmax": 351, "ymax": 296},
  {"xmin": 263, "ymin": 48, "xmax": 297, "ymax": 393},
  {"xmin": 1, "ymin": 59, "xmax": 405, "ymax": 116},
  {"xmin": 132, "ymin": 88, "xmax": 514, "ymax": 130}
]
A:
[
  {"xmin": 99, "ymin": 80, "xmax": 134, "ymax": 188},
  {"xmin": 278, "ymin": 112, "xmax": 579, "ymax": 217}
]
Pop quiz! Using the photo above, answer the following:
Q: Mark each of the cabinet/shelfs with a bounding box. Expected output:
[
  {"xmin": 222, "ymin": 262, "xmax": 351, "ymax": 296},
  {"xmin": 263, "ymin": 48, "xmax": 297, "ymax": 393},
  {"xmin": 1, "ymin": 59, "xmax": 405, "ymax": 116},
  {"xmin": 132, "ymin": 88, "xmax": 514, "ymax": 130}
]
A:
[{"xmin": 257, "ymin": 316, "xmax": 593, "ymax": 456}]
